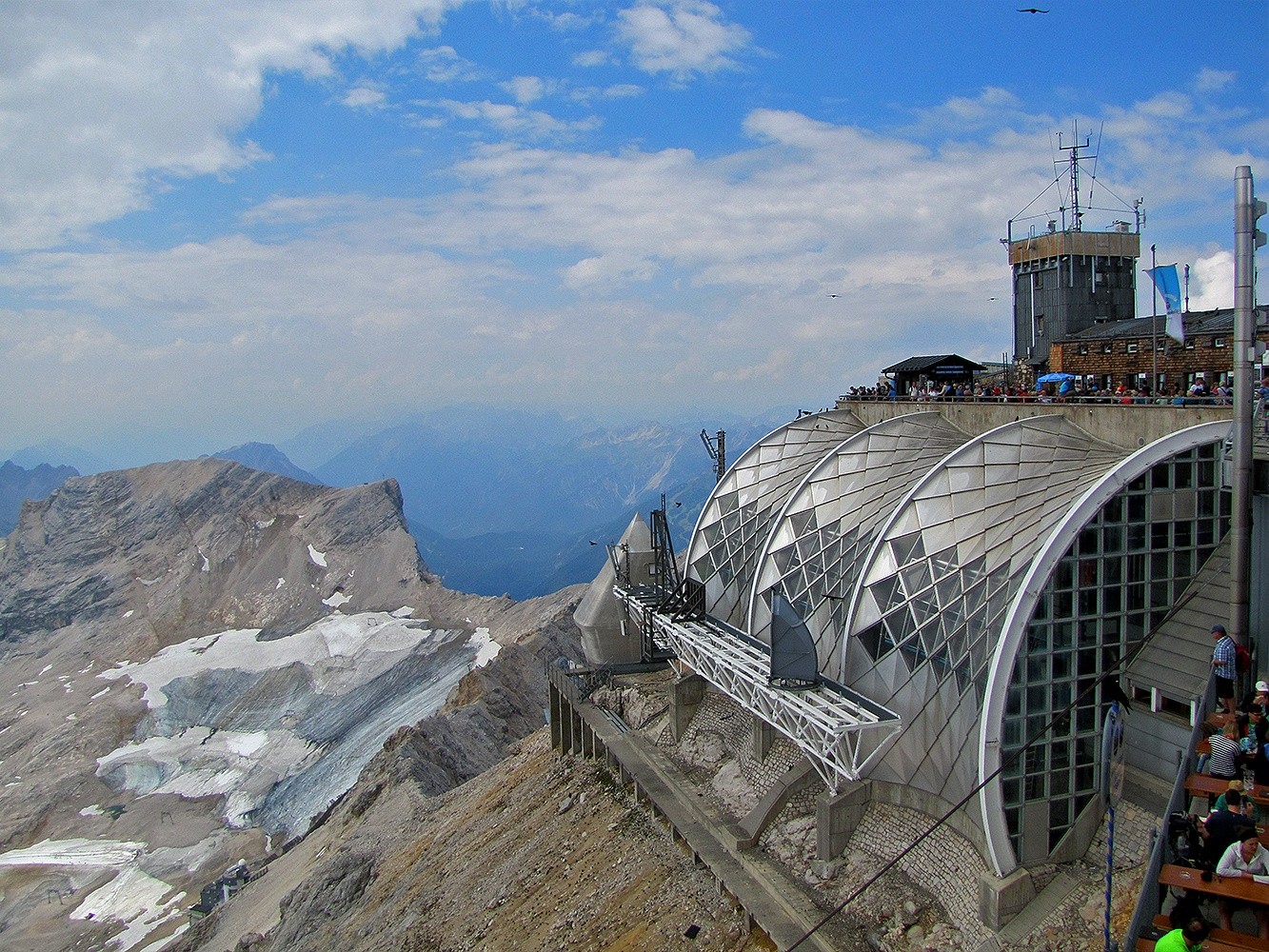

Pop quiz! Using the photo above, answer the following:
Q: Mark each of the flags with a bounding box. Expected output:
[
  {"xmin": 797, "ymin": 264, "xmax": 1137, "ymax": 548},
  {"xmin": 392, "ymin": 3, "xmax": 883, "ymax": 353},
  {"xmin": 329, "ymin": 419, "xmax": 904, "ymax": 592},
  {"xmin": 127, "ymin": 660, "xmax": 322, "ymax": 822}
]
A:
[{"xmin": 1143, "ymin": 263, "xmax": 1185, "ymax": 344}]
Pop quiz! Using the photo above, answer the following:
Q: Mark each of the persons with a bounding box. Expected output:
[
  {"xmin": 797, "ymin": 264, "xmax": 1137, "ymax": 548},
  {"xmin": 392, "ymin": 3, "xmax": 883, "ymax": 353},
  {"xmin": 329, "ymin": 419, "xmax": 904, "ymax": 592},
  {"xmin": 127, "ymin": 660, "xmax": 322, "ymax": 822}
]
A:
[
  {"xmin": 1200, "ymin": 789, "xmax": 1258, "ymax": 868},
  {"xmin": 1214, "ymin": 826, "xmax": 1269, "ymax": 940},
  {"xmin": 1236, "ymin": 680, "xmax": 1269, "ymax": 785},
  {"xmin": 1152, "ymin": 917, "xmax": 1210, "ymax": 952},
  {"xmin": 1207, "ymin": 719, "xmax": 1243, "ymax": 782},
  {"xmin": 1209, "ymin": 623, "xmax": 1239, "ymax": 716},
  {"xmin": 846, "ymin": 363, "xmax": 1269, "ymax": 436},
  {"xmin": 1214, "ymin": 780, "xmax": 1260, "ymax": 823}
]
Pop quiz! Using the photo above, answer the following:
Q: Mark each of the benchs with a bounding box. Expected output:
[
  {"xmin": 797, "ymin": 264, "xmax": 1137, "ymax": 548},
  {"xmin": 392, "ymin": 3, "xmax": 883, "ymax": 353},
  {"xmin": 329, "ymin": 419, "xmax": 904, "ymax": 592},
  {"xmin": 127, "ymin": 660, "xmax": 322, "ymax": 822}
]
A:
[{"xmin": 1133, "ymin": 704, "xmax": 1269, "ymax": 951}]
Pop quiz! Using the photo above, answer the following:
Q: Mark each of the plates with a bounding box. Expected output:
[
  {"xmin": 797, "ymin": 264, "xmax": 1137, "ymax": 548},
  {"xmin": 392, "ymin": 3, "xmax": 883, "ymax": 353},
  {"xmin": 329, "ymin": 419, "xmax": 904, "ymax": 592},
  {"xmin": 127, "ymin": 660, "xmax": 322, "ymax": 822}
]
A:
[
  {"xmin": 1256, "ymin": 827, "xmax": 1265, "ymax": 833},
  {"xmin": 1180, "ymin": 871, "xmax": 1192, "ymax": 879}
]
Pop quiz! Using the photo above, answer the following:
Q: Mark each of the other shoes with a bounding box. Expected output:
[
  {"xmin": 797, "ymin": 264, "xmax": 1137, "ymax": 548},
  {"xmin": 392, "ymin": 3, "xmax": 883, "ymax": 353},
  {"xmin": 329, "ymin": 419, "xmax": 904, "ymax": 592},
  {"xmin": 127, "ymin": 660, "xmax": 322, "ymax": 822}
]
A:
[{"xmin": 1220, "ymin": 708, "xmax": 1230, "ymax": 713}]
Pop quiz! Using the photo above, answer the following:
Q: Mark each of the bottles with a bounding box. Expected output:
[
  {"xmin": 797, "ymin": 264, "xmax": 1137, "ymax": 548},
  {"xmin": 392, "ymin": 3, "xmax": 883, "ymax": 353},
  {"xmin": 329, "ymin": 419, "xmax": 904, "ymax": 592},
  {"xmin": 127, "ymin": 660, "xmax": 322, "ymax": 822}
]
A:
[{"xmin": 1204, "ymin": 933, "xmax": 1211, "ymax": 949}]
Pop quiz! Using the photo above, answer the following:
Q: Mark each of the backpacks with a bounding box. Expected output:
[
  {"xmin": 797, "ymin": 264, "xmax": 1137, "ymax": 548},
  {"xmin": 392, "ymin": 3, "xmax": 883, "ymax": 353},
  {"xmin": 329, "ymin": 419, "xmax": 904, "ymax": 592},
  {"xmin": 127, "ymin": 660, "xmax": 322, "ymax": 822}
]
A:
[
  {"xmin": 1221, "ymin": 388, "xmax": 1234, "ymax": 403},
  {"xmin": 1168, "ymin": 889, "xmax": 1211, "ymax": 933},
  {"xmin": 1169, "ymin": 808, "xmax": 1202, "ymax": 859},
  {"xmin": 1226, "ymin": 635, "xmax": 1250, "ymax": 677}
]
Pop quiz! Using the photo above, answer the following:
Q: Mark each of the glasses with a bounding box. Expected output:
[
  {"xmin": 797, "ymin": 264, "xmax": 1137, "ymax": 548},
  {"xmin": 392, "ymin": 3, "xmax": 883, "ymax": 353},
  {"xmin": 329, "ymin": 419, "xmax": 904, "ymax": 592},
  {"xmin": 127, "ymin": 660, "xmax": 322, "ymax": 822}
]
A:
[{"xmin": 1251, "ymin": 713, "xmax": 1264, "ymax": 716}]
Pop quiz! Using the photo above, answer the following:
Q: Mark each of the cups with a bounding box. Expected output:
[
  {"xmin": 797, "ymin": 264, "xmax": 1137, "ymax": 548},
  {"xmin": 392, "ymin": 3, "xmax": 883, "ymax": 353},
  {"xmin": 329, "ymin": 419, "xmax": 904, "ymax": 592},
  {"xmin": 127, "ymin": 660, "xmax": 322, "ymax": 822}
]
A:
[
  {"xmin": 1243, "ymin": 771, "xmax": 1250, "ymax": 791},
  {"xmin": 1249, "ymin": 770, "xmax": 1254, "ymax": 790},
  {"xmin": 1210, "ymin": 806, "xmax": 1218, "ymax": 815}
]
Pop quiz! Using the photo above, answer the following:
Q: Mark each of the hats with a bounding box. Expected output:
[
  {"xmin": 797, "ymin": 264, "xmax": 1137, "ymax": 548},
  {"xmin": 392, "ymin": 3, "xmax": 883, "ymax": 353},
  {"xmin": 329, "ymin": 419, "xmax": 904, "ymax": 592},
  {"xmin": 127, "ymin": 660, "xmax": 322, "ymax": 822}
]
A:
[
  {"xmin": 1240, "ymin": 829, "xmax": 1260, "ymax": 843},
  {"xmin": 1210, "ymin": 624, "xmax": 1225, "ymax": 633},
  {"xmin": 1256, "ymin": 680, "xmax": 1269, "ymax": 692},
  {"xmin": 1226, "ymin": 780, "xmax": 1248, "ymax": 795}
]
